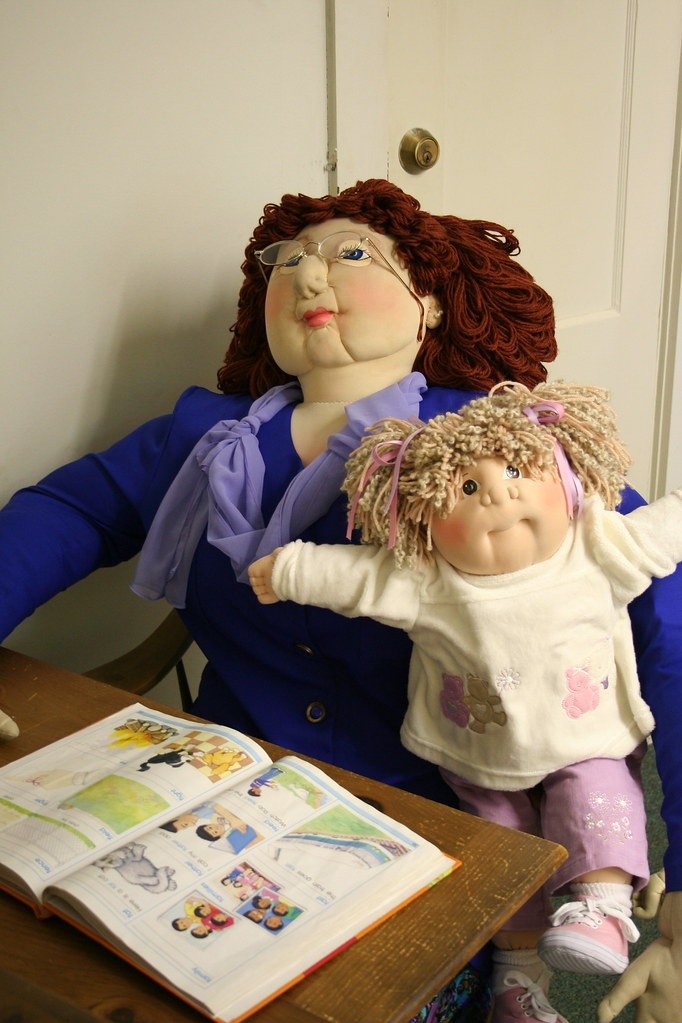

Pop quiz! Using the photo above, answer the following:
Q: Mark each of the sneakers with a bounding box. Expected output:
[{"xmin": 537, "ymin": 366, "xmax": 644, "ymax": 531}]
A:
[
  {"xmin": 538, "ymin": 896, "xmax": 638, "ymax": 974},
  {"xmin": 492, "ymin": 970, "xmax": 569, "ymax": 1023}
]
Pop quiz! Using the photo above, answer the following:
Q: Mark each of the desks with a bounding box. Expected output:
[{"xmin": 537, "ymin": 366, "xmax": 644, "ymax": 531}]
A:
[{"xmin": 1, "ymin": 645, "xmax": 567, "ymax": 1023}]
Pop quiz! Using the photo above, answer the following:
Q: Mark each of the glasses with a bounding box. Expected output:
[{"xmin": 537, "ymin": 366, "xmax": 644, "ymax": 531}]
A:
[{"xmin": 253, "ymin": 230, "xmax": 424, "ymax": 342}]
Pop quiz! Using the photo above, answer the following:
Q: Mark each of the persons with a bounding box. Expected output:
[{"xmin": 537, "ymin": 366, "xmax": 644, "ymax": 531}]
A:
[
  {"xmin": 249, "ymin": 379, "xmax": 681, "ymax": 1022},
  {"xmin": 0, "ymin": 180, "xmax": 681, "ymax": 1023}
]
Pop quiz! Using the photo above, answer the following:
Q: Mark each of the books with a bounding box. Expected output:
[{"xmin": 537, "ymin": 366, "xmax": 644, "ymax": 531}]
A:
[{"xmin": 0, "ymin": 702, "xmax": 463, "ymax": 1023}]
